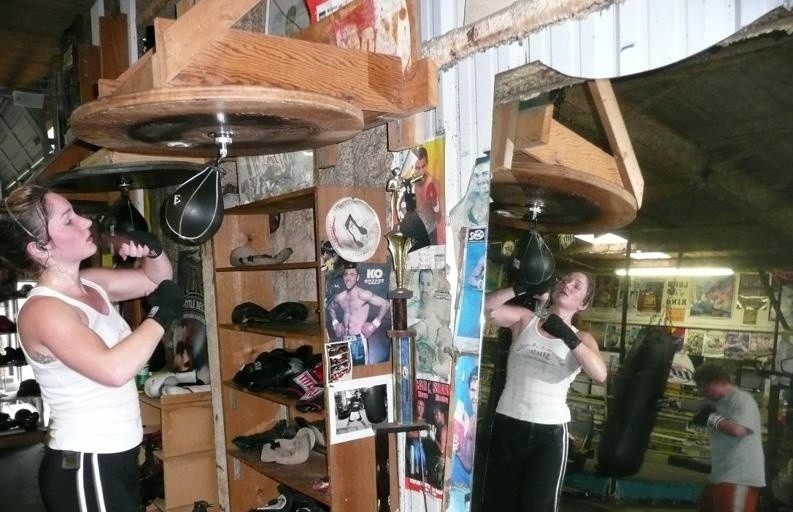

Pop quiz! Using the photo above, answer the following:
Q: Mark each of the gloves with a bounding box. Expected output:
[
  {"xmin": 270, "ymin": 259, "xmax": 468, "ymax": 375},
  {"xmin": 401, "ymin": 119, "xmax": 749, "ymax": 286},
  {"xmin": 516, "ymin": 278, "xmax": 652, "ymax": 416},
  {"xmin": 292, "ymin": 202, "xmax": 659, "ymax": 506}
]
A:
[
  {"xmin": 332, "ymin": 320, "xmax": 344, "ymax": 337},
  {"xmin": 426, "ymin": 183, "xmax": 439, "ymax": 213},
  {"xmin": 362, "ymin": 317, "xmax": 381, "ymax": 339},
  {"xmin": 693, "ymin": 406, "xmax": 724, "ymax": 427},
  {"xmin": 657, "ymin": 396, "xmax": 678, "ymax": 408},
  {"xmin": 229, "ymin": 246, "xmax": 293, "ymax": 266}
]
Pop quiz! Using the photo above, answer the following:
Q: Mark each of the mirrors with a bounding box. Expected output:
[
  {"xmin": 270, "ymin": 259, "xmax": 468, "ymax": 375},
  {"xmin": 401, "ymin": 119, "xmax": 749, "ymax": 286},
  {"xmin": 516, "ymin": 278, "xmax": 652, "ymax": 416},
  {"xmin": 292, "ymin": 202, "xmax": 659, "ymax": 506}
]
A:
[{"xmin": 471, "ymin": 2, "xmax": 793, "ymax": 512}]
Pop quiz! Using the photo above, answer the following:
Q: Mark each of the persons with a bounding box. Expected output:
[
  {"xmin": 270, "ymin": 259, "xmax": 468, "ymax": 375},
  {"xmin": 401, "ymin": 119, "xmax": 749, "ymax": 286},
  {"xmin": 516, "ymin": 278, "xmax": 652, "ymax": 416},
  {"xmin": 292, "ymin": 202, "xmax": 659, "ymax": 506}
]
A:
[
  {"xmin": 449, "ymin": 157, "xmax": 491, "ymax": 243},
  {"xmin": 483, "ymin": 265, "xmax": 608, "ymax": 512},
  {"xmin": 657, "ymin": 363, "xmax": 767, "ymax": 512},
  {"xmin": 167, "ymin": 325, "xmax": 195, "ymax": 374},
  {"xmin": 452, "ymin": 366, "xmax": 479, "ymax": 490},
  {"xmin": 406, "ymin": 269, "xmax": 453, "ymax": 381},
  {"xmin": 388, "ymin": 147, "xmax": 439, "ymax": 246},
  {"xmin": 327, "ymin": 269, "xmax": 390, "ymax": 366},
  {"xmin": 0, "ymin": 182, "xmax": 186, "ymax": 512},
  {"xmin": 407, "ymin": 397, "xmax": 447, "ymax": 491},
  {"xmin": 473, "ymin": 254, "xmax": 487, "ymax": 329}
]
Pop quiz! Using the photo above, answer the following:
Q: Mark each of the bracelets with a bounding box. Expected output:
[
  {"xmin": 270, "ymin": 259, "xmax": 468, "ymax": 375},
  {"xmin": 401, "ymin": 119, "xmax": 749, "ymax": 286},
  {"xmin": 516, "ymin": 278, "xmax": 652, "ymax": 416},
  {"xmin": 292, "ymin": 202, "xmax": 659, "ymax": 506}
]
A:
[
  {"xmin": 372, "ymin": 318, "xmax": 381, "ymax": 330},
  {"xmin": 331, "ymin": 319, "xmax": 339, "ymax": 327}
]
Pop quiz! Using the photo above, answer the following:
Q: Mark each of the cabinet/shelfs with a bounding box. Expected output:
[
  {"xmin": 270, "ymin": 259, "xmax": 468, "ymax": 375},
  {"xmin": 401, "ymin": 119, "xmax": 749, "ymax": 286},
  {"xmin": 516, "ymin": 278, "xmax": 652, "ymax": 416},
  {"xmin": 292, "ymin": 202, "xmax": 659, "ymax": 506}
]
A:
[
  {"xmin": 139, "ymin": 185, "xmax": 403, "ymax": 511},
  {"xmin": 482, "ymin": 336, "xmax": 793, "ymax": 459}
]
